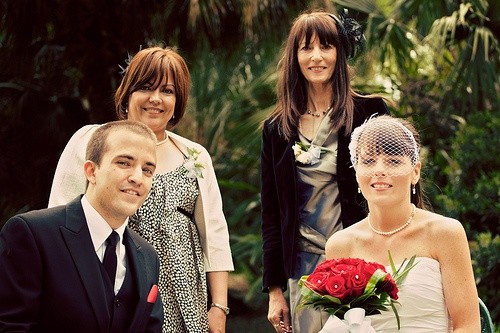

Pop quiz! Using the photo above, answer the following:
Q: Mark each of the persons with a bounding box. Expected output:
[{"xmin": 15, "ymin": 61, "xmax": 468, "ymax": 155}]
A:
[
  {"xmin": 0, "ymin": 118, "xmax": 165, "ymax": 333},
  {"xmin": 48, "ymin": 46, "xmax": 235, "ymax": 333},
  {"xmin": 260, "ymin": 10, "xmax": 393, "ymax": 333},
  {"xmin": 324, "ymin": 114, "xmax": 482, "ymax": 333}
]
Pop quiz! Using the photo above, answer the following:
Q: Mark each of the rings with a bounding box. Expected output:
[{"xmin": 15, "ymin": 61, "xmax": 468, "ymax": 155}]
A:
[{"xmin": 274, "ymin": 324, "xmax": 281, "ymax": 327}]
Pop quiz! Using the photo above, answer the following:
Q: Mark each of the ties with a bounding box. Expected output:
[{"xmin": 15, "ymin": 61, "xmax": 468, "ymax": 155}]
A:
[{"xmin": 102, "ymin": 231, "xmax": 120, "ymax": 290}]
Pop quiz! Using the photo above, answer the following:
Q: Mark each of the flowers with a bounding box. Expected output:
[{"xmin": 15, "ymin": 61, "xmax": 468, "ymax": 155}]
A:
[
  {"xmin": 293, "ymin": 142, "xmax": 326, "ymax": 167},
  {"xmin": 292, "ymin": 250, "xmax": 421, "ymax": 333},
  {"xmin": 182, "ymin": 147, "xmax": 204, "ymax": 179},
  {"xmin": 340, "ymin": 9, "xmax": 366, "ymax": 58}
]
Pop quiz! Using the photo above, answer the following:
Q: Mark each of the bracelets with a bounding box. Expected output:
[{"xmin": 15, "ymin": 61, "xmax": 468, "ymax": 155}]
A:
[{"xmin": 211, "ymin": 303, "xmax": 230, "ymax": 315}]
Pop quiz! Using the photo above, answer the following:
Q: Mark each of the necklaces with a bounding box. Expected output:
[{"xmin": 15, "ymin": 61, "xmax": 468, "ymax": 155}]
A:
[
  {"xmin": 305, "ymin": 103, "xmax": 331, "ymax": 118},
  {"xmin": 156, "ymin": 132, "xmax": 168, "ymax": 145},
  {"xmin": 366, "ymin": 201, "xmax": 416, "ymax": 235}
]
